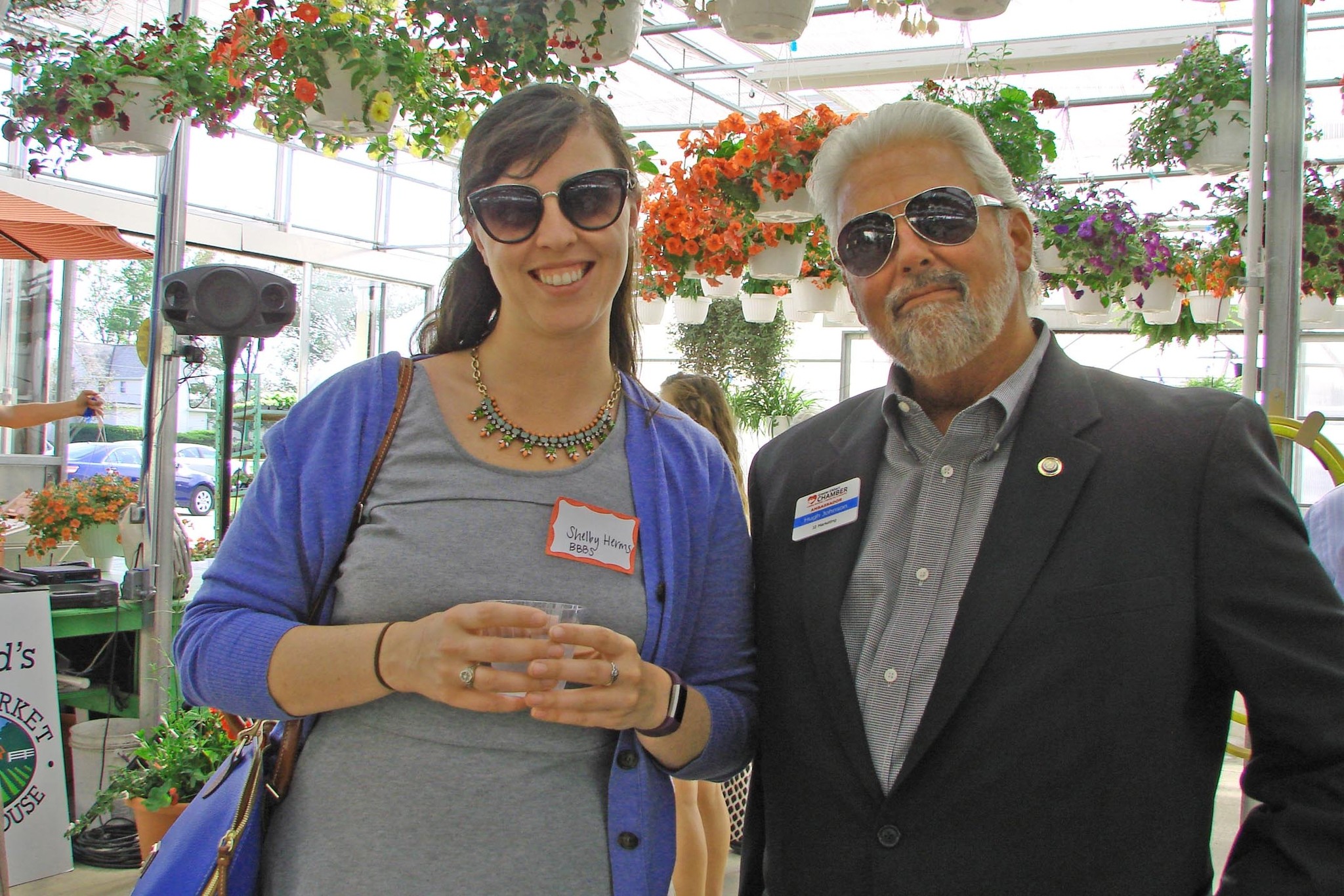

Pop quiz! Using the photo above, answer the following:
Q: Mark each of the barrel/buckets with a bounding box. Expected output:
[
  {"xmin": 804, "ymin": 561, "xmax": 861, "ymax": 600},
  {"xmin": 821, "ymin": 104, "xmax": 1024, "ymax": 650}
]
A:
[{"xmin": 68, "ymin": 716, "xmax": 143, "ymax": 830}]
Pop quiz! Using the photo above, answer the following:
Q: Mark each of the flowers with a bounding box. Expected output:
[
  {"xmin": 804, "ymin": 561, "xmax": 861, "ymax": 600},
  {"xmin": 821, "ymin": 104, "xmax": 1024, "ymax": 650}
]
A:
[
  {"xmin": 0, "ymin": 0, "xmax": 1344, "ymax": 340},
  {"xmin": 19, "ymin": 467, "xmax": 139, "ymax": 560}
]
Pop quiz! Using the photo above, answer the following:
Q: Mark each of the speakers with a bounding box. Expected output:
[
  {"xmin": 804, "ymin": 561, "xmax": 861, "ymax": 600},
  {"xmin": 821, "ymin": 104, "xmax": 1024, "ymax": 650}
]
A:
[{"xmin": 161, "ymin": 263, "xmax": 298, "ymax": 339}]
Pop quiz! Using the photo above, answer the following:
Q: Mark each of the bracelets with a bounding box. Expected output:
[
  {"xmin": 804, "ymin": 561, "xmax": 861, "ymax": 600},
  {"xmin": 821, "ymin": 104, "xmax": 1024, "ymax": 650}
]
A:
[{"xmin": 375, "ymin": 621, "xmax": 398, "ymax": 691}]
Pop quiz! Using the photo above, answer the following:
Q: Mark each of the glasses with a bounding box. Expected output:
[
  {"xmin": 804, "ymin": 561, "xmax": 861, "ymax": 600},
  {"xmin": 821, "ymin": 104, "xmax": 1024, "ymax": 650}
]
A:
[
  {"xmin": 830, "ymin": 185, "xmax": 1013, "ymax": 279},
  {"xmin": 466, "ymin": 167, "xmax": 634, "ymax": 245}
]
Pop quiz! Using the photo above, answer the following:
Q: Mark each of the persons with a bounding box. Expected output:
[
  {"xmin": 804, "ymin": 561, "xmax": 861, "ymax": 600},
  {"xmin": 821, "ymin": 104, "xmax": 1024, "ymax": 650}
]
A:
[
  {"xmin": 738, "ymin": 102, "xmax": 1343, "ymax": 894},
  {"xmin": 173, "ymin": 82, "xmax": 755, "ymax": 894}
]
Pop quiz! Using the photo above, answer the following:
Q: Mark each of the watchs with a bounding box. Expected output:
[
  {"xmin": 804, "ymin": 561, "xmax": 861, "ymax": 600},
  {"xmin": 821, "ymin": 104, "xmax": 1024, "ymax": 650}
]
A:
[{"xmin": 633, "ymin": 666, "xmax": 688, "ymax": 737}]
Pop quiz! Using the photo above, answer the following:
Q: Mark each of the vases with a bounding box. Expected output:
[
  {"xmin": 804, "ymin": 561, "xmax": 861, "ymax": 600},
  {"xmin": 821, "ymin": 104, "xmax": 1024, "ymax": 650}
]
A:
[
  {"xmin": 1060, "ymin": 279, "xmax": 1112, "ymax": 316},
  {"xmin": 749, "ymin": 233, "xmax": 805, "ymax": 278},
  {"xmin": 300, "ymin": 46, "xmax": 407, "ymax": 136},
  {"xmin": 739, "ymin": 293, "xmax": 779, "ymax": 322},
  {"xmin": 1122, "ymin": 273, "xmax": 1179, "ymax": 312},
  {"xmin": 78, "ymin": 517, "xmax": 123, "ymax": 557},
  {"xmin": 749, "ymin": 167, "xmax": 816, "ymax": 223},
  {"xmin": 92, "ymin": 76, "xmax": 183, "ymax": 156},
  {"xmin": 1033, "ymin": 233, "xmax": 1080, "ymax": 274},
  {"xmin": 542, "ymin": 0, "xmax": 644, "ymax": 68},
  {"xmin": 1186, "ymin": 289, "xmax": 1231, "ymax": 324},
  {"xmin": 716, "ymin": 0, "xmax": 815, "ymax": 43},
  {"xmin": 789, "ymin": 276, "xmax": 839, "ymax": 312},
  {"xmin": 1142, "ymin": 292, "xmax": 1184, "ymax": 325},
  {"xmin": 1168, "ymin": 100, "xmax": 1250, "ymax": 175},
  {"xmin": 699, "ymin": 267, "xmax": 745, "ymax": 299}
]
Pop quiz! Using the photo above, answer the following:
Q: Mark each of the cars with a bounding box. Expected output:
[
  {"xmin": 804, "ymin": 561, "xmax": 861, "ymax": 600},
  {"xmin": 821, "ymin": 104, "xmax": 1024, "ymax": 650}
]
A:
[{"xmin": 45, "ymin": 441, "xmax": 249, "ymax": 517}]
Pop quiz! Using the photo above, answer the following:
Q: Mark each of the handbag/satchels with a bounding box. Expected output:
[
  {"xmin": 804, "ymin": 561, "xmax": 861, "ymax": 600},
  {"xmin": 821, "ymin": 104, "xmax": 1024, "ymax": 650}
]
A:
[{"xmin": 126, "ymin": 712, "xmax": 298, "ymax": 896}]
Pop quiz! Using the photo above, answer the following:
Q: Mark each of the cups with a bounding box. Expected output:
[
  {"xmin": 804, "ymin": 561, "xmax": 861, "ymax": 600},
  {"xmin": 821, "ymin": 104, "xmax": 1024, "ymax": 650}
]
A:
[{"xmin": 481, "ymin": 599, "xmax": 583, "ymax": 700}]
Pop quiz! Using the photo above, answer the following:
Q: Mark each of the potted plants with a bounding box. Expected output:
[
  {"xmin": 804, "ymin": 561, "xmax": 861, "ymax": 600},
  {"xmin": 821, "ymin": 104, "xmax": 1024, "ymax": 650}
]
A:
[{"xmin": 61, "ymin": 638, "xmax": 238, "ymax": 867}]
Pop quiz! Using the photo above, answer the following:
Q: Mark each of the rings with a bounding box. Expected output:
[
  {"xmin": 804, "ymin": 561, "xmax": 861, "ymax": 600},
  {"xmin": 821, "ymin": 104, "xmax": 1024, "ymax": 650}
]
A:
[
  {"xmin": 604, "ymin": 658, "xmax": 621, "ymax": 688},
  {"xmin": 459, "ymin": 663, "xmax": 478, "ymax": 691}
]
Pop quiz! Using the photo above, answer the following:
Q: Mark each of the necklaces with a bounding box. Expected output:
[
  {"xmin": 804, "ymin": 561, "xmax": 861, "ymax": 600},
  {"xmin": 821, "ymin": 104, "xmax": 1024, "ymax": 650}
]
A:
[{"xmin": 468, "ymin": 342, "xmax": 620, "ymax": 464}]
